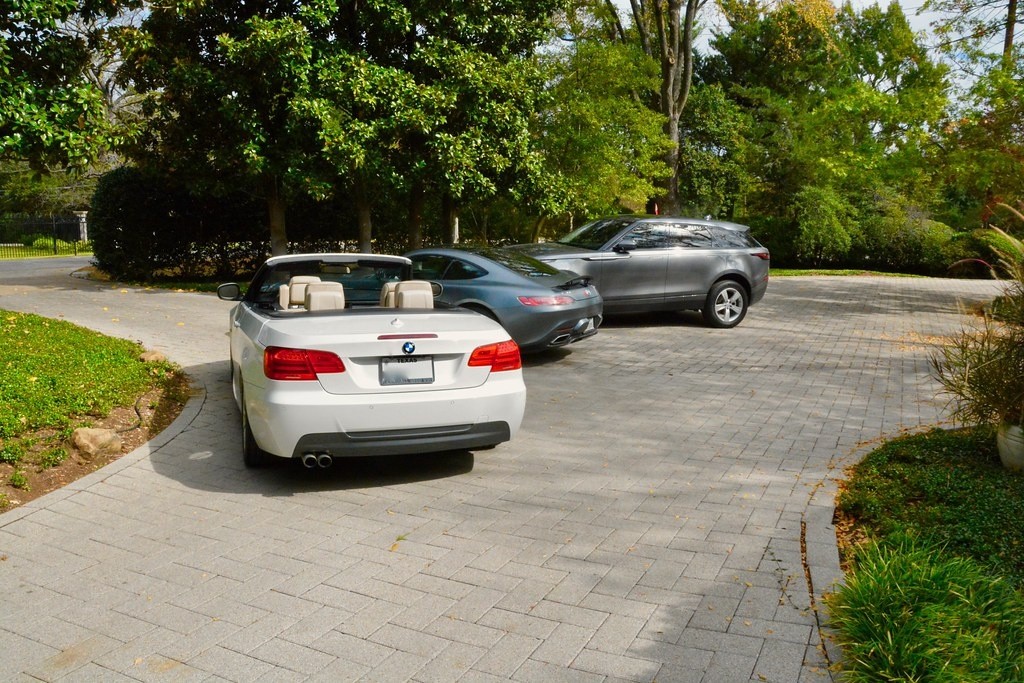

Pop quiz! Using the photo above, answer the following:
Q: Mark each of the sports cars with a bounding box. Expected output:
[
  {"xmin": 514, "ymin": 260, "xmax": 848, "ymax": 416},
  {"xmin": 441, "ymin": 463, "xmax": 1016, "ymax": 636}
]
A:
[{"xmin": 216, "ymin": 253, "xmax": 526, "ymax": 469}]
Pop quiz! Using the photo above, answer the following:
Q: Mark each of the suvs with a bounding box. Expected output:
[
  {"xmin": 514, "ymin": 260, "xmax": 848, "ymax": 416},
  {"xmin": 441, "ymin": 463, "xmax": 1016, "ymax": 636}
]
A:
[{"xmin": 502, "ymin": 215, "xmax": 770, "ymax": 329}]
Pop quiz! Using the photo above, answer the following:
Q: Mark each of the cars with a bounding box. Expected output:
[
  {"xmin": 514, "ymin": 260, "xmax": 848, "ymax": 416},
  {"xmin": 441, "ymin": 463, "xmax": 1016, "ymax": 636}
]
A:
[{"xmin": 338, "ymin": 248, "xmax": 604, "ymax": 354}]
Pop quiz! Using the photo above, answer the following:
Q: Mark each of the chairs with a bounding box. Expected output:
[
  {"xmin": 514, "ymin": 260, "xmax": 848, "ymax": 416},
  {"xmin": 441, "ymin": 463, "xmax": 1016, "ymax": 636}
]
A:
[
  {"xmin": 272, "ymin": 275, "xmax": 322, "ymax": 310},
  {"xmin": 379, "ymin": 279, "xmax": 435, "ymax": 310},
  {"xmin": 277, "ymin": 281, "xmax": 347, "ymax": 313}
]
